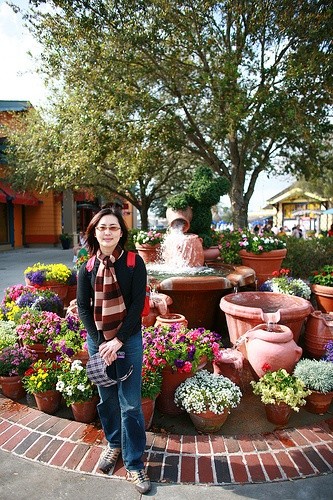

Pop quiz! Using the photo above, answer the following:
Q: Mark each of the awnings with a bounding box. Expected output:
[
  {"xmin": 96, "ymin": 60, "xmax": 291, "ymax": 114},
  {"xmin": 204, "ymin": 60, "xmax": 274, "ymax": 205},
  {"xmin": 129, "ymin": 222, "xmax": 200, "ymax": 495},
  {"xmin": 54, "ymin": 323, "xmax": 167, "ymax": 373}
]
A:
[{"xmin": 0, "ymin": 181, "xmax": 41, "ymax": 206}]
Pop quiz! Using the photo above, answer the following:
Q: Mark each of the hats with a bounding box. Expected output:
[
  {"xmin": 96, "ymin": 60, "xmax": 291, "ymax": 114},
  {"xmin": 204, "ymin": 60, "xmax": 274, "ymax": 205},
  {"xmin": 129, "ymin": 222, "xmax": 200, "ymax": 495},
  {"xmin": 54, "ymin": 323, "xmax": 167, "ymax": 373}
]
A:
[{"xmin": 86, "ymin": 351, "xmax": 134, "ymax": 387}]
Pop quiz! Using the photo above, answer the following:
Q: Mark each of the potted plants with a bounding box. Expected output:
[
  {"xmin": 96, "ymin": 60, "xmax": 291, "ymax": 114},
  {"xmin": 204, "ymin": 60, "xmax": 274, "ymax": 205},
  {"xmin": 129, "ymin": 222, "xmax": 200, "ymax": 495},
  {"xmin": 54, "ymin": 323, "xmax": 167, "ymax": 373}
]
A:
[{"xmin": 60, "ymin": 233, "xmax": 72, "ymax": 249}]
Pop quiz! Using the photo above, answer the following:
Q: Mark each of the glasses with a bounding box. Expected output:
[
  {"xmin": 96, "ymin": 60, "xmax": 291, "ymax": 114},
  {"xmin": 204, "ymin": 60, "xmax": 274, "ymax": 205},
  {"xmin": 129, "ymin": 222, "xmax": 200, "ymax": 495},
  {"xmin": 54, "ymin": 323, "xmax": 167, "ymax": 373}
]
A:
[{"xmin": 95, "ymin": 226, "xmax": 122, "ymax": 231}]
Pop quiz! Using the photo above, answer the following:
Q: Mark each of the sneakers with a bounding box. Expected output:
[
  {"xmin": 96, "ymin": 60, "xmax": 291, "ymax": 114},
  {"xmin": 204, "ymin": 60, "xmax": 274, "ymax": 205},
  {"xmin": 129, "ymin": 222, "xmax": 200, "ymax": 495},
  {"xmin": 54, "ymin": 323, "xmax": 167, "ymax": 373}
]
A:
[
  {"xmin": 127, "ymin": 468, "xmax": 151, "ymax": 494},
  {"xmin": 99, "ymin": 443, "xmax": 122, "ymax": 473}
]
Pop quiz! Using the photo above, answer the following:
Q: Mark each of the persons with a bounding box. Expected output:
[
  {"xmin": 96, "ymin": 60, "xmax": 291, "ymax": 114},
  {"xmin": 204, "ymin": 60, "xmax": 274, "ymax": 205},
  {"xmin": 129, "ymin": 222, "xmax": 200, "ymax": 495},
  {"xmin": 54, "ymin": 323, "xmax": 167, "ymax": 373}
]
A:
[
  {"xmin": 329, "ymin": 224, "xmax": 333, "ymax": 237},
  {"xmin": 73, "ymin": 231, "xmax": 85, "ymax": 262},
  {"xmin": 77, "ymin": 208, "xmax": 151, "ymax": 494},
  {"xmin": 254, "ymin": 224, "xmax": 306, "ymax": 239}
]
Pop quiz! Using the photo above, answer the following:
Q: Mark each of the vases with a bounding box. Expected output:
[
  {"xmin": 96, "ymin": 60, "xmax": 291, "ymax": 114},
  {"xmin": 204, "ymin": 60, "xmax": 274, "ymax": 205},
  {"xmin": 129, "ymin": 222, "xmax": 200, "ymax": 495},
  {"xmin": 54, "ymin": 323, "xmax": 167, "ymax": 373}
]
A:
[
  {"xmin": 34, "ymin": 390, "xmax": 61, "ymax": 414},
  {"xmin": 0, "ymin": 376, "xmax": 27, "ymax": 400},
  {"xmin": 64, "ymin": 285, "xmax": 77, "ymax": 306},
  {"xmin": 30, "ymin": 279, "xmax": 68, "ymax": 309},
  {"xmin": 75, "ymin": 350, "xmax": 89, "ymax": 366},
  {"xmin": 27, "ymin": 343, "xmax": 57, "ymax": 361},
  {"xmin": 141, "ymin": 207, "xmax": 333, "ymax": 434},
  {"xmin": 72, "ymin": 397, "xmax": 97, "ymax": 422}
]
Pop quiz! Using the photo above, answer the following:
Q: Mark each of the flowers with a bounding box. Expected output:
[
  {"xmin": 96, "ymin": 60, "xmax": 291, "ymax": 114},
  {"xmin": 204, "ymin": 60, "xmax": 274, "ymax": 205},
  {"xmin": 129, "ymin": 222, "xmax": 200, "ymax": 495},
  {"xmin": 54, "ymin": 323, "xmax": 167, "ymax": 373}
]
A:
[
  {"xmin": 260, "ymin": 277, "xmax": 310, "ymax": 292},
  {"xmin": 250, "ymin": 368, "xmax": 312, "ymax": 412},
  {"xmin": 320, "ymin": 340, "xmax": 333, "ymax": 362},
  {"xmin": 0, "ymin": 222, "xmax": 251, "ymax": 415},
  {"xmin": 218, "ymin": 227, "xmax": 286, "ymax": 264},
  {"xmin": 270, "ymin": 269, "xmax": 311, "ymax": 300},
  {"xmin": 310, "ymin": 265, "xmax": 333, "ymax": 287},
  {"xmin": 293, "ymin": 357, "xmax": 333, "ymax": 395}
]
